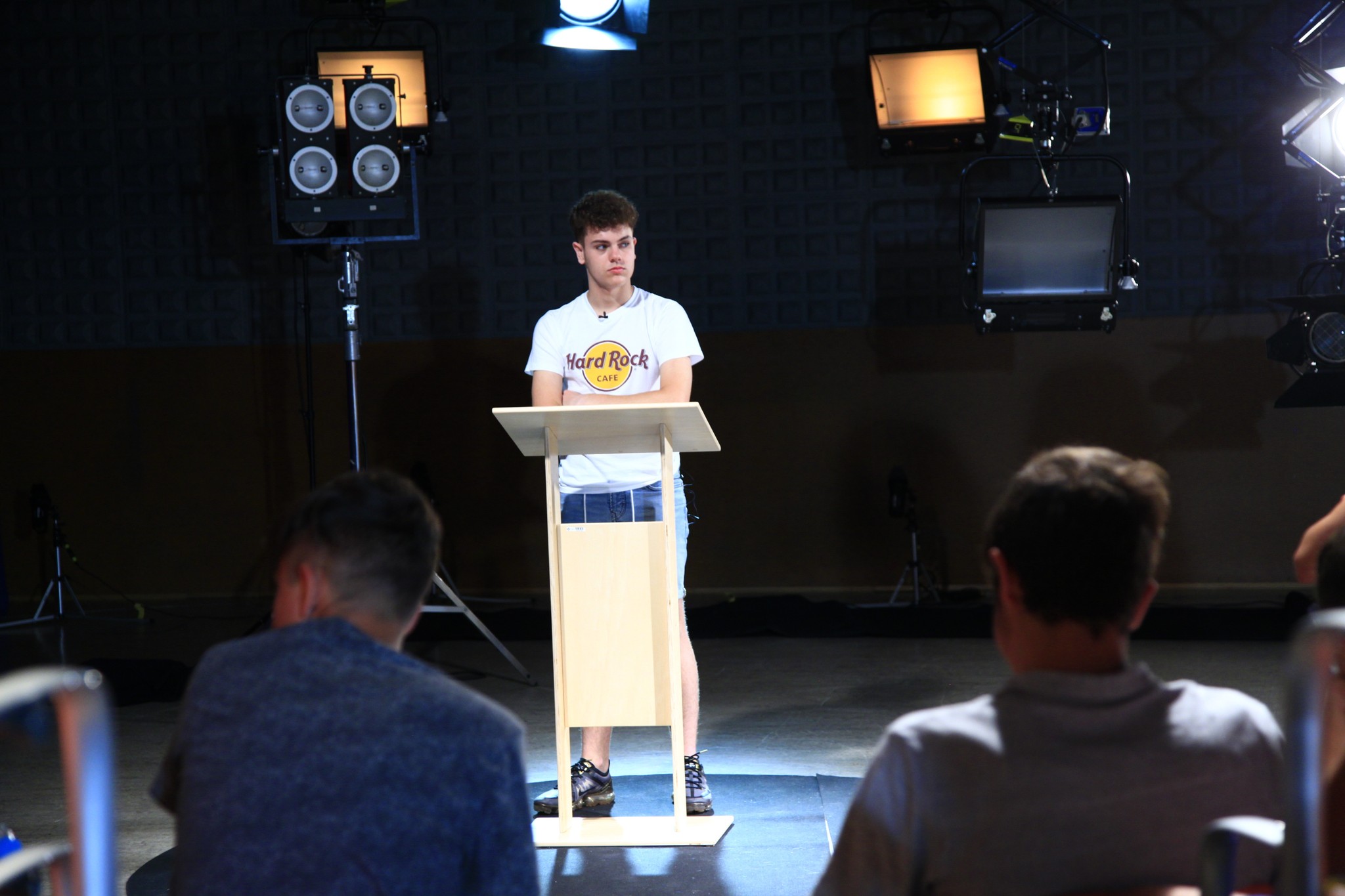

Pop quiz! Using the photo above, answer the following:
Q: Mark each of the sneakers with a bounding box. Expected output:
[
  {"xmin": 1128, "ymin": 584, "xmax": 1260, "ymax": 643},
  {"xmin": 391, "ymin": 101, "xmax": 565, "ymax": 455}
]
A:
[
  {"xmin": 671, "ymin": 749, "xmax": 713, "ymax": 813},
  {"xmin": 533, "ymin": 757, "xmax": 615, "ymax": 813}
]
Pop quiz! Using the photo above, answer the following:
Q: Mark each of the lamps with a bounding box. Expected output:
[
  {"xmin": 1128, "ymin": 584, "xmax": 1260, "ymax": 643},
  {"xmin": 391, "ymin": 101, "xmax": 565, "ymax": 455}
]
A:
[
  {"xmin": 1279, "ymin": 1, "xmax": 1345, "ymax": 191},
  {"xmin": 960, "ymin": 152, "xmax": 1141, "ymax": 337},
  {"xmin": 1269, "ymin": 254, "xmax": 1345, "ymax": 410},
  {"xmin": 866, "ymin": 2, "xmax": 1004, "ymax": 132},
  {"xmin": 540, "ymin": 0, "xmax": 651, "ymax": 49},
  {"xmin": 310, "ymin": 42, "xmax": 439, "ymax": 154},
  {"xmin": 258, "ymin": 66, "xmax": 537, "ymax": 688}
]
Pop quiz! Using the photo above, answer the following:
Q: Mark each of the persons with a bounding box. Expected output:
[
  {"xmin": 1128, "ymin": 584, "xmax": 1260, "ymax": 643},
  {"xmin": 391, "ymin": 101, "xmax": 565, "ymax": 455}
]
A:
[
  {"xmin": 523, "ymin": 190, "xmax": 714, "ymax": 815},
  {"xmin": 812, "ymin": 448, "xmax": 1284, "ymax": 896},
  {"xmin": 146, "ymin": 466, "xmax": 541, "ymax": 896},
  {"xmin": 1295, "ymin": 486, "xmax": 1345, "ymax": 611}
]
